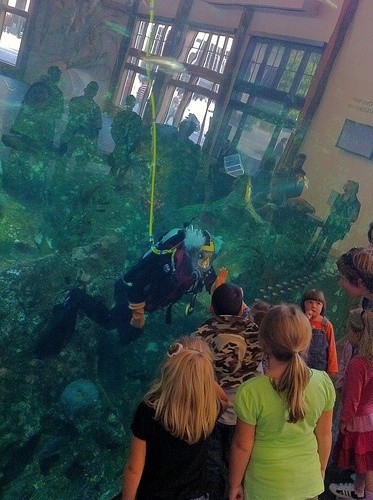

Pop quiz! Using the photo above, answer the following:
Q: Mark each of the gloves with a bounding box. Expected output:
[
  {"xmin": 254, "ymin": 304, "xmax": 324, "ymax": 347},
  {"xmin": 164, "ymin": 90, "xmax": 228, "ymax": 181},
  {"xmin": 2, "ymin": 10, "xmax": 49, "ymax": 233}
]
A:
[
  {"xmin": 129, "ymin": 302, "xmax": 145, "ymax": 328},
  {"xmin": 215, "ymin": 267, "xmax": 228, "ymax": 287}
]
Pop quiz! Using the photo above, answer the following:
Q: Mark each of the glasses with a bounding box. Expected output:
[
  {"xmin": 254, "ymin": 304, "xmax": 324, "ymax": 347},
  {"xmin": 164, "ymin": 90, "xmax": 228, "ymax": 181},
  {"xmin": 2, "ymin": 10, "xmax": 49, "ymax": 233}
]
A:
[{"xmin": 199, "ymin": 252, "xmax": 215, "ymax": 261}]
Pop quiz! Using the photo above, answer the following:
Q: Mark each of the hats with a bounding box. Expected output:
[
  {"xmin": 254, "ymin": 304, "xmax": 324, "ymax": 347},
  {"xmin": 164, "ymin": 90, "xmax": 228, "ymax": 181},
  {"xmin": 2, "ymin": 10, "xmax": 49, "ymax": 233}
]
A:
[
  {"xmin": 196, "ymin": 315, "xmax": 263, "ymax": 389},
  {"xmin": 201, "ymin": 230, "xmax": 214, "ymax": 252}
]
